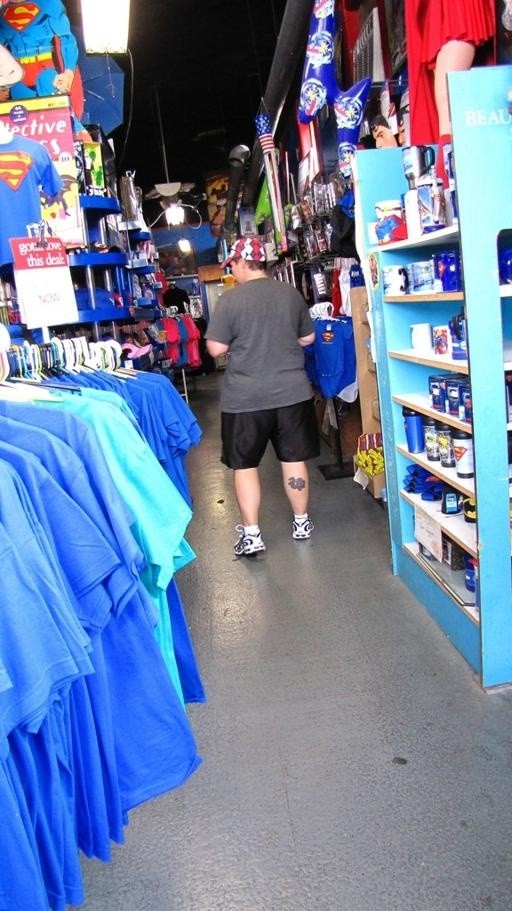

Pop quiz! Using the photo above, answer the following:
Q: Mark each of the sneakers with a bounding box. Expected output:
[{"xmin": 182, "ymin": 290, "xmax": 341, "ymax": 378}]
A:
[
  {"xmin": 292, "ymin": 518, "xmax": 315, "ymax": 540},
  {"xmin": 234, "ymin": 526, "xmax": 266, "ymax": 556}
]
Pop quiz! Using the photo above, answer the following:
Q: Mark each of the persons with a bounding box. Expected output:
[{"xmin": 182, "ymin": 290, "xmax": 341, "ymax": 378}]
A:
[
  {"xmin": 204, "ymin": 237, "xmax": 320, "ymax": 556},
  {"xmin": 397, "ymin": 111, "xmax": 410, "ymax": 147},
  {"xmin": 419, "ymin": 0, "xmax": 496, "ymax": 144},
  {"xmin": 370, "ymin": 114, "xmax": 399, "ymax": 148},
  {"xmin": 0, "ymin": 0, "xmax": 79, "ymax": 101},
  {"xmin": 0, "ymin": 121, "xmax": 64, "ymax": 270},
  {"xmin": 375, "ymin": 207, "xmax": 398, "ymax": 245}
]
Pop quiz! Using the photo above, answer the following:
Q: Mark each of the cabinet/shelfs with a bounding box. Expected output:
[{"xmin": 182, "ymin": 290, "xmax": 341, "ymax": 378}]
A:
[
  {"xmin": 62, "ymin": 194, "xmax": 172, "ymax": 377},
  {"xmin": 348, "ymin": 68, "xmax": 512, "ymax": 695}
]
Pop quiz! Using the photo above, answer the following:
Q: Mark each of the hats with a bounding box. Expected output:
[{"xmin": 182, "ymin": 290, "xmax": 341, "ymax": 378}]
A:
[
  {"xmin": 121, "ymin": 342, "xmax": 152, "ymax": 360},
  {"xmin": 219, "ymin": 237, "xmax": 266, "ymax": 269}
]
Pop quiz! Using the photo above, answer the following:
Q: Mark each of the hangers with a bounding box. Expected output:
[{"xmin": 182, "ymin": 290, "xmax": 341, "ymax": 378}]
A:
[
  {"xmin": 308, "ymin": 301, "xmax": 350, "ymax": 325},
  {"xmin": 1, "ymin": 338, "xmax": 166, "ymax": 425},
  {"xmin": 161, "ymin": 305, "xmax": 194, "ymax": 325}
]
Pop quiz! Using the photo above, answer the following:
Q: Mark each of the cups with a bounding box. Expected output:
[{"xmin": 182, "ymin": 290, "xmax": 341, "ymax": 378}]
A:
[
  {"xmin": 437, "ymin": 424, "xmax": 455, "ymax": 468},
  {"xmin": 402, "ymin": 409, "xmax": 424, "ymax": 454},
  {"xmin": 452, "ymin": 429, "xmax": 475, "ymax": 478},
  {"xmin": 415, "ymin": 174, "xmax": 446, "ymax": 233},
  {"xmin": 422, "ymin": 417, "xmax": 440, "ymax": 462}
]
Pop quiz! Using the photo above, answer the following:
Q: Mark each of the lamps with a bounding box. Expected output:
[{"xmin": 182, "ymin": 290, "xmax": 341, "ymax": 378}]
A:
[{"xmin": 154, "ymin": 81, "xmax": 182, "ymax": 196}]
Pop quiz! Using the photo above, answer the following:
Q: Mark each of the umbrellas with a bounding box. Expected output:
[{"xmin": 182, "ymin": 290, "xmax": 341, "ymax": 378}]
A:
[{"xmin": 78, "ymin": 56, "xmax": 125, "ymax": 137}]
